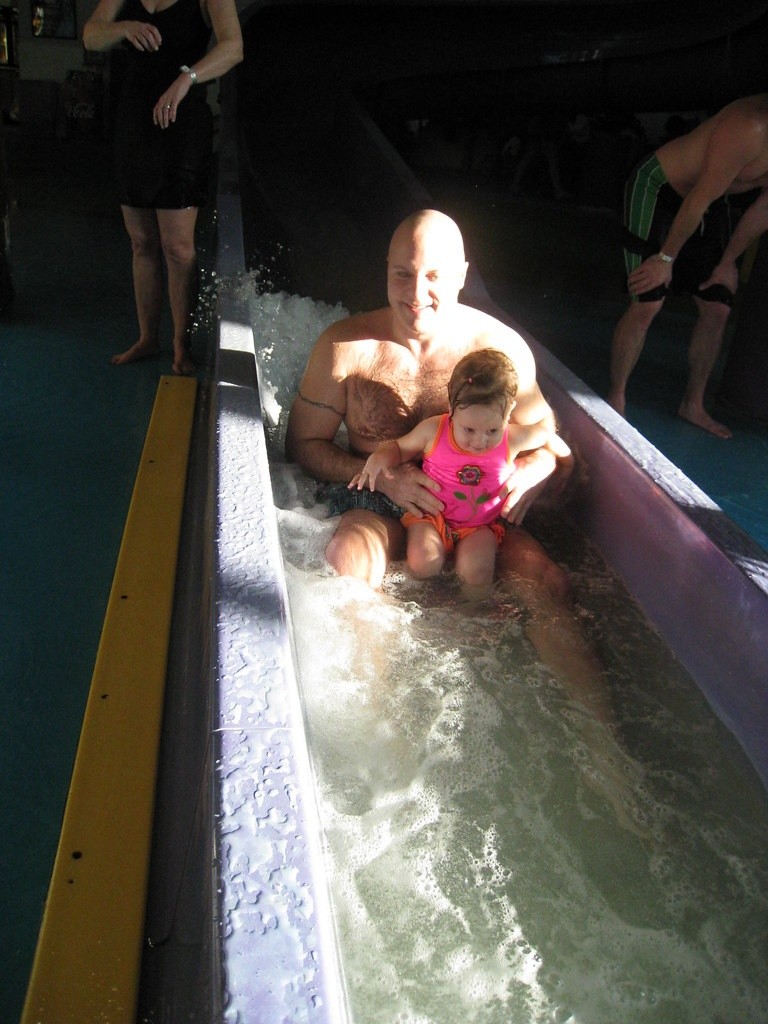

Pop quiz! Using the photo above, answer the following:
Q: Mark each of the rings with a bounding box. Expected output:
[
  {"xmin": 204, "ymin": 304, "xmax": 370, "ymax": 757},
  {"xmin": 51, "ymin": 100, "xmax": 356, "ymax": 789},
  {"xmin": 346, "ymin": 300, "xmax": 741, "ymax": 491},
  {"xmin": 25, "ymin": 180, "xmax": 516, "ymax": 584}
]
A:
[{"xmin": 165, "ymin": 104, "xmax": 170, "ymax": 109}]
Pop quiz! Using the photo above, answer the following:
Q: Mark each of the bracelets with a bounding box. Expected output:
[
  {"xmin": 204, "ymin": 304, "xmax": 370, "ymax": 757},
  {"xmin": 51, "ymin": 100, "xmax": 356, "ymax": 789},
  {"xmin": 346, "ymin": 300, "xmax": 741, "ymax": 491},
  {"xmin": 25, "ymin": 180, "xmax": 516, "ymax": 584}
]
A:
[
  {"xmin": 180, "ymin": 65, "xmax": 198, "ymax": 84},
  {"xmin": 659, "ymin": 251, "xmax": 676, "ymax": 264}
]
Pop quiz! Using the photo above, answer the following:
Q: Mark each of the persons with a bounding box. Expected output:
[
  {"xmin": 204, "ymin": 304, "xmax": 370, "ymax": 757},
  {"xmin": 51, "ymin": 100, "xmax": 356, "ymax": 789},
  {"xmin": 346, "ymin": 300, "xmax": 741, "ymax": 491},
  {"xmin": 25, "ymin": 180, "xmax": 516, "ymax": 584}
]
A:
[
  {"xmin": 346, "ymin": 348, "xmax": 576, "ymax": 585},
  {"xmin": 283, "ymin": 208, "xmax": 664, "ymax": 586},
  {"xmin": 82, "ymin": 0, "xmax": 244, "ymax": 375},
  {"xmin": 603, "ymin": 90, "xmax": 768, "ymax": 441}
]
